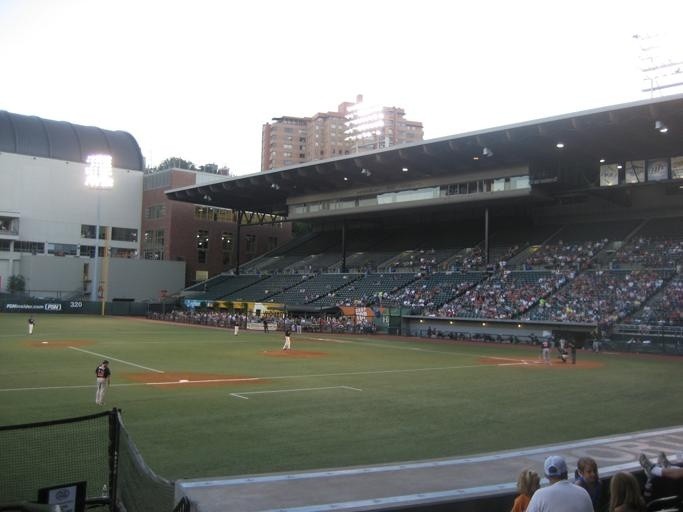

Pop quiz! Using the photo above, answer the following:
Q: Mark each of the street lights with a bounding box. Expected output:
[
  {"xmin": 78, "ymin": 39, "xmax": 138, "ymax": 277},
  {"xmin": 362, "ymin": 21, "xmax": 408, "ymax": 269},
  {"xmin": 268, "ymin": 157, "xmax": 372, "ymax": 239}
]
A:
[
  {"xmin": 344, "ymin": 101, "xmax": 385, "ymax": 155},
  {"xmin": 85, "ymin": 153, "xmax": 114, "ymax": 301},
  {"xmin": 634, "ymin": 30, "xmax": 682, "ymax": 96}
]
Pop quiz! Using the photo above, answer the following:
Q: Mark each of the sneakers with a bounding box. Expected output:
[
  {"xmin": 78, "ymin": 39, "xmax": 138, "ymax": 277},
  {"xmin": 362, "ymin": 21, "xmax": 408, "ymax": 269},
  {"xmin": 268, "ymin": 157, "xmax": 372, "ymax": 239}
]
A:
[
  {"xmin": 640, "ymin": 454, "xmax": 655, "ymax": 478},
  {"xmin": 658, "ymin": 452, "xmax": 671, "ymax": 469}
]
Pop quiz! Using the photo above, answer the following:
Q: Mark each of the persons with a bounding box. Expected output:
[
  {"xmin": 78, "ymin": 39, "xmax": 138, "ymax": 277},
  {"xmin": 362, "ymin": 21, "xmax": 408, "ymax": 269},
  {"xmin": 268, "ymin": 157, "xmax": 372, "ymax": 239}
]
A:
[
  {"xmin": 297, "ymin": 315, "xmax": 376, "ymax": 335},
  {"xmin": 574, "ymin": 457, "xmax": 607, "ymax": 511},
  {"xmin": 428, "ymin": 326, "xmax": 432, "ymax": 336},
  {"xmin": 511, "ymin": 468, "xmax": 541, "ymax": 511},
  {"xmin": 432, "ymin": 237, "xmax": 682, "ymax": 337},
  {"xmin": 281, "ymin": 329, "xmax": 292, "ymax": 350},
  {"xmin": 541, "ymin": 338, "xmax": 551, "ymax": 364},
  {"xmin": 525, "ymin": 455, "xmax": 595, "ymax": 511},
  {"xmin": 301, "ymin": 247, "xmax": 437, "ymax": 308},
  {"xmin": 608, "ymin": 470, "xmax": 647, "ymax": 511},
  {"xmin": 639, "ymin": 451, "xmax": 682, "ymax": 480},
  {"xmin": 567, "ymin": 340, "xmax": 577, "ymax": 364},
  {"xmin": 199, "ymin": 317, "xmax": 214, "ymax": 319},
  {"xmin": 27, "ymin": 315, "xmax": 35, "ymax": 335},
  {"xmin": 147, "ymin": 306, "xmax": 296, "ymax": 339},
  {"xmin": 591, "ymin": 331, "xmax": 600, "ymax": 354},
  {"xmin": 94, "ymin": 360, "xmax": 111, "ymax": 408}
]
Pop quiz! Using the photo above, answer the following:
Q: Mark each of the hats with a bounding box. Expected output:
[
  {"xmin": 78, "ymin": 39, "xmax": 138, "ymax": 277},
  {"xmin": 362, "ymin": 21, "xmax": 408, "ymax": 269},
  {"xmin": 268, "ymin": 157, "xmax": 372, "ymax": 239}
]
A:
[{"xmin": 544, "ymin": 455, "xmax": 567, "ymax": 476}]
